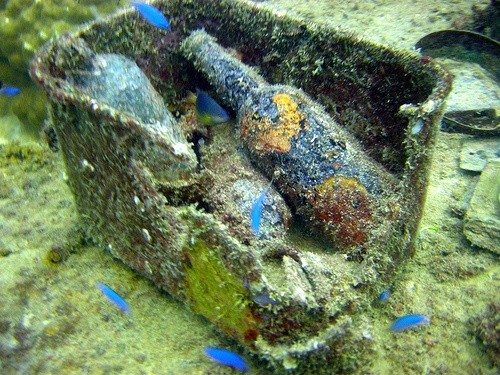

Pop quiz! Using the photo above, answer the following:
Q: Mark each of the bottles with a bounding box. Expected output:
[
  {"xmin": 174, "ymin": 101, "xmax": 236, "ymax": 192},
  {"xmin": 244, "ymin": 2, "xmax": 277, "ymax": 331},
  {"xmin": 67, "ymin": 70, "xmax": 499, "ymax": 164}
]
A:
[{"xmin": 178, "ymin": 27, "xmax": 407, "ymax": 259}]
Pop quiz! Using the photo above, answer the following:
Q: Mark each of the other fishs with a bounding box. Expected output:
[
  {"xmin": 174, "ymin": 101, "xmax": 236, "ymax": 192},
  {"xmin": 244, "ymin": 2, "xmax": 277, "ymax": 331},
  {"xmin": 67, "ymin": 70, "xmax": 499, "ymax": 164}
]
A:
[
  {"xmin": 181, "ymin": 89, "xmax": 228, "ymax": 124},
  {"xmin": 251, "ymin": 175, "xmax": 273, "ymax": 235},
  {"xmin": 203, "ymin": 346, "xmax": 249, "ymax": 371},
  {"xmin": 379, "ymin": 291, "xmax": 388, "ymax": 300},
  {"xmin": 131, "ymin": 1, "xmax": 171, "ymax": 31},
  {"xmin": 96, "ymin": 281, "xmax": 131, "ymax": 313},
  {"xmin": 0, "ymin": 87, "xmax": 22, "ymax": 96},
  {"xmin": 389, "ymin": 314, "xmax": 430, "ymax": 331}
]
